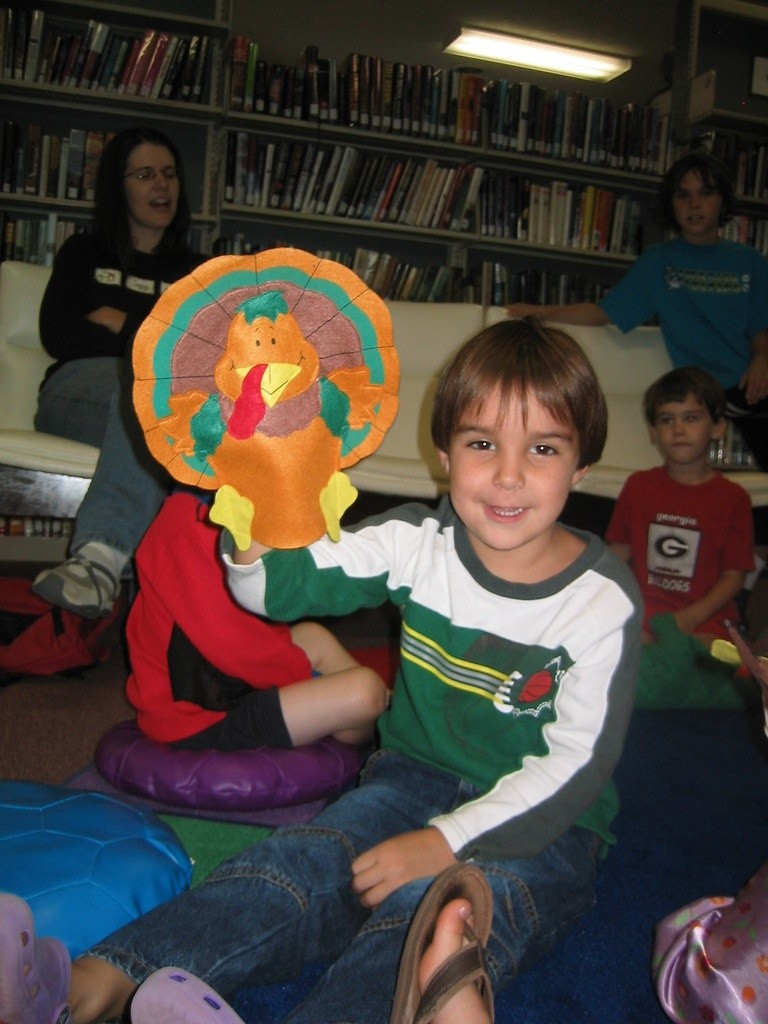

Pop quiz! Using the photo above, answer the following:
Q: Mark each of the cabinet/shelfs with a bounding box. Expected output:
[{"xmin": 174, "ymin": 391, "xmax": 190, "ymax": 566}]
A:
[{"xmin": 0, "ymin": 0, "xmax": 767, "ymax": 574}]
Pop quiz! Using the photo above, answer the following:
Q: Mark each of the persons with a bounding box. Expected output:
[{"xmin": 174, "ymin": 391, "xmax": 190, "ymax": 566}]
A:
[{"xmin": 0, "ymin": 126, "xmax": 768, "ymax": 1024}]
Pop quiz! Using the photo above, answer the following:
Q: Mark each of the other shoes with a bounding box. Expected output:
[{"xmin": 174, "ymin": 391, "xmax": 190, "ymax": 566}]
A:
[{"xmin": 32, "ymin": 545, "xmax": 122, "ymax": 620}]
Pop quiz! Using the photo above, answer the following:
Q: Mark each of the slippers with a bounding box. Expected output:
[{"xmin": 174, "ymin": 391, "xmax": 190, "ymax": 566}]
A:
[
  {"xmin": 0, "ymin": 893, "xmax": 71, "ymax": 1024},
  {"xmin": 131, "ymin": 966, "xmax": 246, "ymax": 1024},
  {"xmin": 388, "ymin": 862, "xmax": 495, "ymax": 1024}
]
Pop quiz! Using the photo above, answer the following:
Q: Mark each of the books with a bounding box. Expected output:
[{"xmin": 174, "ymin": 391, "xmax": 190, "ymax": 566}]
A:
[{"xmin": 0, "ymin": 0, "xmax": 768, "ymax": 535}]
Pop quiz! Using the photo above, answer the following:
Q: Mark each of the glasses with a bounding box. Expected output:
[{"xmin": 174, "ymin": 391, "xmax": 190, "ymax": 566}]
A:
[{"xmin": 122, "ymin": 166, "xmax": 179, "ymax": 183}]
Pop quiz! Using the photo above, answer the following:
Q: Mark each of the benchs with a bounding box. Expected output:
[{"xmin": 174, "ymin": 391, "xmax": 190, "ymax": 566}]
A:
[{"xmin": 0, "ymin": 258, "xmax": 768, "ymax": 531}]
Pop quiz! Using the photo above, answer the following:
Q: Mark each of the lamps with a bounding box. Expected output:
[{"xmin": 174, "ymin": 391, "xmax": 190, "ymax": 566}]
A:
[{"xmin": 439, "ymin": 26, "xmax": 632, "ymax": 82}]
